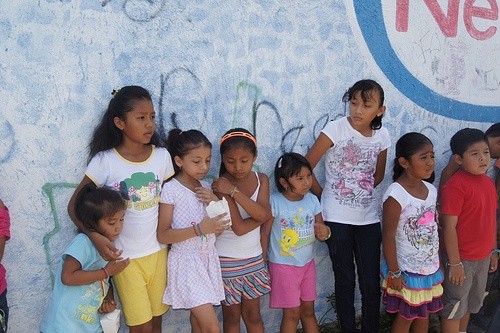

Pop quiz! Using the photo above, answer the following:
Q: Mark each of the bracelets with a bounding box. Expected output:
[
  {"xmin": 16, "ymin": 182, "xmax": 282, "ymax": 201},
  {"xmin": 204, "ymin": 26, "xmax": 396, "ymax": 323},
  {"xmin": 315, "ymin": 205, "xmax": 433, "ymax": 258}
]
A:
[
  {"xmin": 325, "ymin": 226, "xmax": 331, "ymax": 240},
  {"xmin": 491, "ymin": 249, "xmax": 500, "ymax": 254},
  {"xmin": 447, "ymin": 262, "xmax": 464, "ymax": 268},
  {"xmin": 387, "ymin": 267, "xmax": 402, "ymax": 280},
  {"xmin": 191, "ymin": 222, "xmax": 202, "ymax": 236},
  {"xmin": 101, "ymin": 268, "xmax": 108, "ymax": 279}
]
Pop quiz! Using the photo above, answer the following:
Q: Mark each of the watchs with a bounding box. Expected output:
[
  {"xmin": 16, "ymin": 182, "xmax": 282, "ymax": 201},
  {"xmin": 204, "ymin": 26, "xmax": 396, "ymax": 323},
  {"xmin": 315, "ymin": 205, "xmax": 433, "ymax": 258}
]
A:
[{"xmin": 231, "ymin": 186, "xmax": 240, "ymax": 197}]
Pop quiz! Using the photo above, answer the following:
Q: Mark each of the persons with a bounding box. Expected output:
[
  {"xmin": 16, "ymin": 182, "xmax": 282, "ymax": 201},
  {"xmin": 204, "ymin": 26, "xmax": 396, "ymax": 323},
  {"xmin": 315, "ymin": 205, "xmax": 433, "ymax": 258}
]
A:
[
  {"xmin": 260, "ymin": 152, "xmax": 332, "ymax": 332},
  {"xmin": 37, "ymin": 182, "xmax": 128, "ymax": 333},
  {"xmin": 0, "ymin": 199, "xmax": 11, "ymax": 333},
  {"xmin": 380, "ymin": 132, "xmax": 446, "ymax": 333},
  {"xmin": 303, "ymin": 78, "xmax": 392, "ymax": 333},
  {"xmin": 157, "ymin": 128, "xmax": 233, "ymax": 333},
  {"xmin": 439, "ymin": 121, "xmax": 499, "ymax": 193},
  {"xmin": 67, "ymin": 84, "xmax": 176, "ymax": 333},
  {"xmin": 210, "ymin": 127, "xmax": 272, "ymax": 333},
  {"xmin": 439, "ymin": 127, "xmax": 500, "ymax": 333},
  {"xmin": 474, "ymin": 158, "xmax": 500, "ymax": 319}
]
